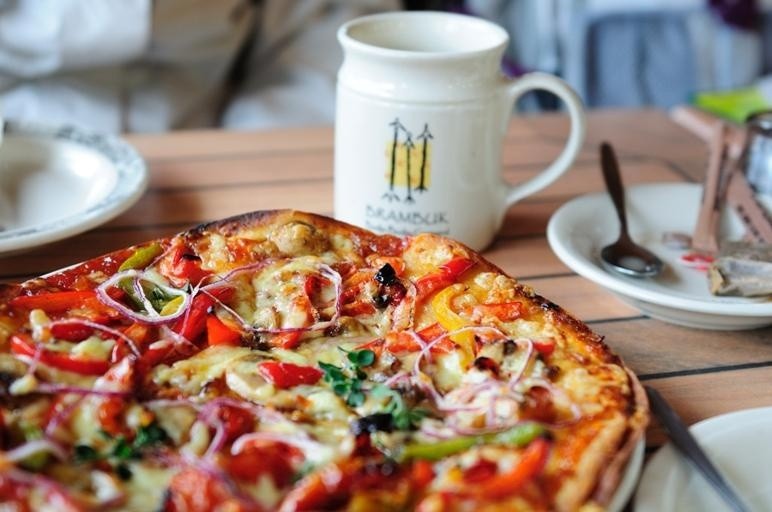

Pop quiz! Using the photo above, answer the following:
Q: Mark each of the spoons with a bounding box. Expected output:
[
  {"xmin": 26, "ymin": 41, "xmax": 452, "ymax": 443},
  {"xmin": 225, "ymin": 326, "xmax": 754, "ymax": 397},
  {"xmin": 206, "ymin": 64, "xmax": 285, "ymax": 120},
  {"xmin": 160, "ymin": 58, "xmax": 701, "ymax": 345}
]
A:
[{"xmin": 595, "ymin": 142, "xmax": 662, "ymax": 278}]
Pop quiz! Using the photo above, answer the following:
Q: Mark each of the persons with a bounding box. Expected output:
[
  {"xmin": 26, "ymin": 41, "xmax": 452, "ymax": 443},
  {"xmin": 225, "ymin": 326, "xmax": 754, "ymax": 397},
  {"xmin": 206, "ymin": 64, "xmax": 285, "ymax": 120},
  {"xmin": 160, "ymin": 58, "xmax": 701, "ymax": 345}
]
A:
[{"xmin": 0, "ymin": 0, "xmax": 407, "ymax": 137}]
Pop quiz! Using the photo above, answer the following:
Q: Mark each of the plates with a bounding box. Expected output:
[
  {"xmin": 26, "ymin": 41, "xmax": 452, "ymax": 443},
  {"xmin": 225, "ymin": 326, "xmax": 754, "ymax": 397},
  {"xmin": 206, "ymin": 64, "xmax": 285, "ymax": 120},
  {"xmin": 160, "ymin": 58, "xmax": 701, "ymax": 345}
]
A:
[
  {"xmin": 548, "ymin": 182, "xmax": 772, "ymax": 330},
  {"xmin": 2, "ymin": 116, "xmax": 149, "ymax": 258},
  {"xmin": 630, "ymin": 405, "xmax": 770, "ymax": 510}
]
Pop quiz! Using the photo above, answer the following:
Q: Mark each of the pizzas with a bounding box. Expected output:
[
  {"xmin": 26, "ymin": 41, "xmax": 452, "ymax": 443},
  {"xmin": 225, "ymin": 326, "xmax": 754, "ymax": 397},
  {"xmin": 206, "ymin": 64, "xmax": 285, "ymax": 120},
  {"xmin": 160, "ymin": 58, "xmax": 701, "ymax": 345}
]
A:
[{"xmin": 0, "ymin": 206, "xmax": 650, "ymax": 512}]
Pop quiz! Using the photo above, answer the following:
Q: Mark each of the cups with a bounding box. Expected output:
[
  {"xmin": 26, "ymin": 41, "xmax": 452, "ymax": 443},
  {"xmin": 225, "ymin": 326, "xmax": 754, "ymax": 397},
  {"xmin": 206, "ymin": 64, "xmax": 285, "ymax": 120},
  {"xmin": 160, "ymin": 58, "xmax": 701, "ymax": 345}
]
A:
[{"xmin": 334, "ymin": 12, "xmax": 585, "ymax": 248}]
